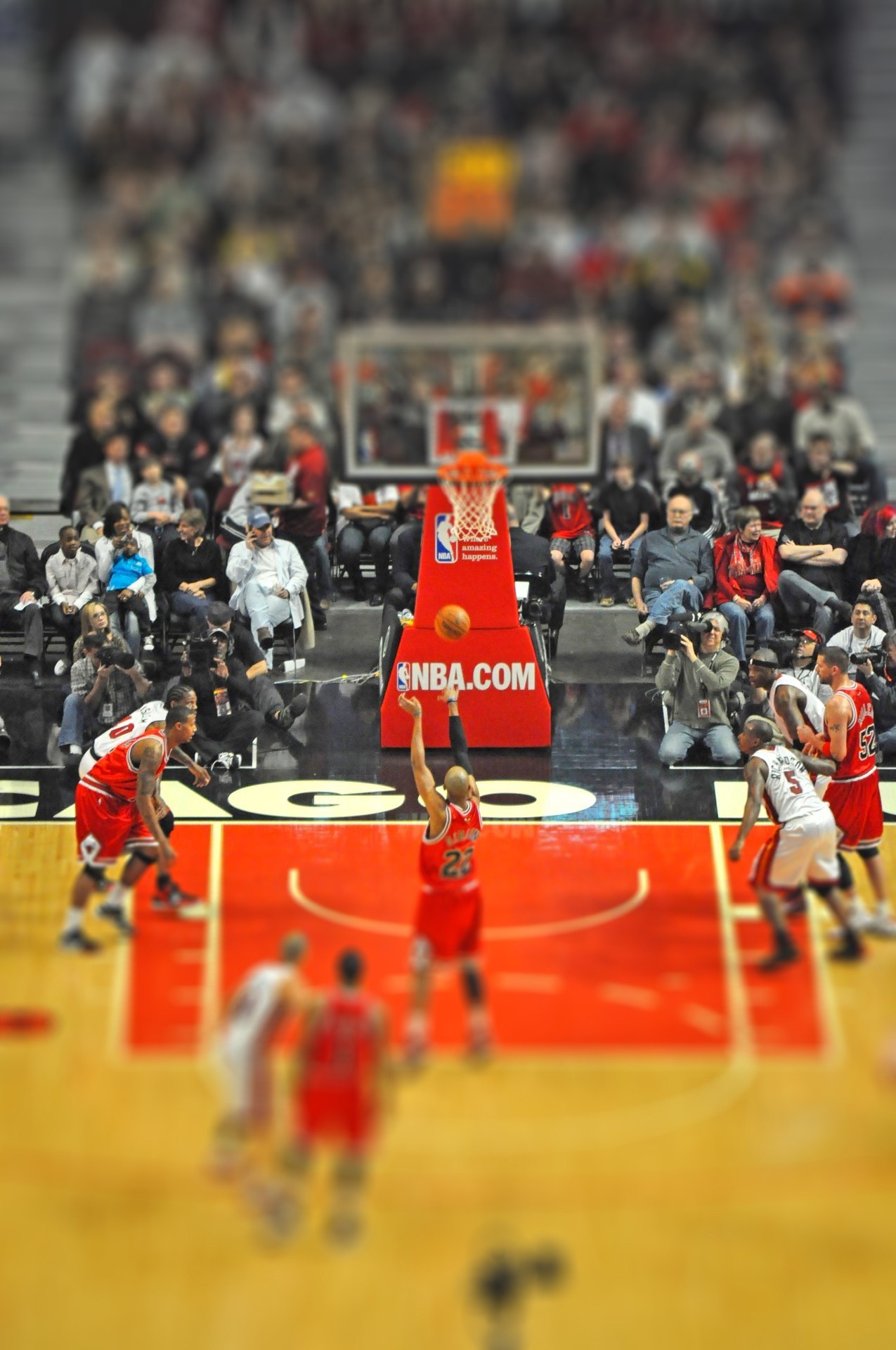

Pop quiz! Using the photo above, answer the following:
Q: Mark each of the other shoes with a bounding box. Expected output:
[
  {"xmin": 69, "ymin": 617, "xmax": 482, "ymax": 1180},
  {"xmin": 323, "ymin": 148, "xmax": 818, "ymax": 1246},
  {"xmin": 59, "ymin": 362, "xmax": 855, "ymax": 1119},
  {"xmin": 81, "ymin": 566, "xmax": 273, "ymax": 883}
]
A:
[
  {"xmin": 831, "ymin": 944, "xmax": 865, "ymax": 960},
  {"xmin": 621, "ymin": 628, "xmax": 642, "ymax": 646},
  {"xmin": 839, "ymin": 600, "xmax": 855, "ymax": 622},
  {"xmin": 565, "ymin": 576, "xmax": 592, "ymax": 603},
  {"xmin": 277, "ymin": 692, "xmax": 306, "ymax": 728},
  {"xmin": 32, "ymin": 669, "xmax": 44, "ymax": 688},
  {"xmin": 759, "ymin": 944, "xmax": 794, "ymax": 969},
  {"xmin": 99, "ymin": 906, "xmax": 136, "ymax": 935},
  {"xmin": 152, "ymin": 877, "xmax": 196, "ymax": 913},
  {"xmin": 60, "ymin": 929, "xmax": 99, "ymax": 952},
  {"xmin": 63, "ymin": 753, "xmax": 82, "ymax": 772}
]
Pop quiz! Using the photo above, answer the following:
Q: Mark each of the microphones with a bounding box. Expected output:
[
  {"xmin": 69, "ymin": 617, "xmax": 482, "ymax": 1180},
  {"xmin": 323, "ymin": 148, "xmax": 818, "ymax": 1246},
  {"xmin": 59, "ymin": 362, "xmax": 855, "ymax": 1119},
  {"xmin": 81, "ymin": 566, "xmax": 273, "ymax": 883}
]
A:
[
  {"xmin": 667, "ymin": 613, "xmax": 701, "ymax": 623},
  {"xmin": 800, "ymin": 655, "xmax": 813, "ymax": 660}
]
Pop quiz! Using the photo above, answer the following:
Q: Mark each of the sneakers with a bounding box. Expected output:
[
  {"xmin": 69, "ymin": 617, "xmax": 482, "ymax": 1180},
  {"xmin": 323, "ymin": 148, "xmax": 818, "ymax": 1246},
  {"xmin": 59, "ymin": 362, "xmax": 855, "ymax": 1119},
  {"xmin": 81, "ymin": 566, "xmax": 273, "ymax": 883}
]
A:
[
  {"xmin": 143, "ymin": 635, "xmax": 155, "ymax": 650},
  {"xmin": 600, "ymin": 594, "xmax": 619, "ymax": 606},
  {"xmin": 54, "ymin": 659, "xmax": 70, "ymax": 675},
  {"xmin": 211, "ymin": 752, "xmax": 239, "ymax": 772},
  {"xmin": 621, "ymin": 589, "xmax": 636, "ymax": 607},
  {"xmin": 194, "ymin": 752, "xmax": 204, "ymax": 767}
]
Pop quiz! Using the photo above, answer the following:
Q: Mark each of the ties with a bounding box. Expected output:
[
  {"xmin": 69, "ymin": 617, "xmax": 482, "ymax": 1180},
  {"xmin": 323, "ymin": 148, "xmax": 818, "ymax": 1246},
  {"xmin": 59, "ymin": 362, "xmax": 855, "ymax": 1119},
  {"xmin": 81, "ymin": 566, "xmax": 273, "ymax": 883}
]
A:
[{"xmin": 112, "ymin": 465, "xmax": 124, "ymax": 503}]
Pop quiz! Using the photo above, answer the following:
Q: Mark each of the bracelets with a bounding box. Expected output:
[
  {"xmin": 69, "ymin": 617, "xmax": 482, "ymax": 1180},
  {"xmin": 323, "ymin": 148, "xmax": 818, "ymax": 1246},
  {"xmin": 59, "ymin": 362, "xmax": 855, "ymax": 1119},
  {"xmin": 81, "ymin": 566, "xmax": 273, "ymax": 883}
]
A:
[
  {"xmin": 734, "ymin": 596, "xmax": 740, "ymax": 603},
  {"xmin": 447, "ymin": 698, "xmax": 457, "ymax": 703},
  {"xmin": 414, "ymin": 711, "xmax": 421, "ymax": 715}
]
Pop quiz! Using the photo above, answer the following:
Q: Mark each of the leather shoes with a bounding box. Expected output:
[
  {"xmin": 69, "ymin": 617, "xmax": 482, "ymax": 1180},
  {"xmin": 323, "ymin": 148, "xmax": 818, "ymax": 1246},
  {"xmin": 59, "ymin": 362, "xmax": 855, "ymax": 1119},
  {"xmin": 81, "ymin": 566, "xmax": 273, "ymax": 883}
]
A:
[
  {"xmin": 353, "ymin": 580, "xmax": 369, "ymax": 601},
  {"xmin": 369, "ymin": 586, "xmax": 384, "ymax": 606}
]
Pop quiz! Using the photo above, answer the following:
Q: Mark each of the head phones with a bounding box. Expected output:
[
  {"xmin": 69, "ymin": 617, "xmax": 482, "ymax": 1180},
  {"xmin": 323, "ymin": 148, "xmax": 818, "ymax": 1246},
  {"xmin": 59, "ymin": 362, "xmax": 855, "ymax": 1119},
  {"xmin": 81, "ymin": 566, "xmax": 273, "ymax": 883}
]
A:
[
  {"xmin": 205, "ymin": 628, "xmax": 234, "ymax": 655},
  {"xmin": 797, "ymin": 627, "xmax": 824, "ymax": 660}
]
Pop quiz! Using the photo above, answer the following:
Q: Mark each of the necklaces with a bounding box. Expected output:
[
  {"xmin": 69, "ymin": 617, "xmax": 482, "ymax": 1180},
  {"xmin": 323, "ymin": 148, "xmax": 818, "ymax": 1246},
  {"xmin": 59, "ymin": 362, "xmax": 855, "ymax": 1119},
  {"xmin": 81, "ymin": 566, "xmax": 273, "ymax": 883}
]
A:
[{"xmin": 848, "ymin": 631, "xmax": 872, "ymax": 671}]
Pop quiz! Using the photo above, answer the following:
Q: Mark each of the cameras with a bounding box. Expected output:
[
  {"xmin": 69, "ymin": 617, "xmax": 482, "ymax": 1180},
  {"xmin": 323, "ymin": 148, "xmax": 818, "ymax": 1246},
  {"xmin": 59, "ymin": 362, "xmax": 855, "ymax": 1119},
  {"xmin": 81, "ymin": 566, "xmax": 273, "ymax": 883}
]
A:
[
  {"xmin": 95, "ymin": 647, "xmax": 135, "ymax": 669},
  {"xmin": 522, "ymin": 598, "xmax": 551, "ymax": 623},
  {"xmin": 850, "ymin": 647, "xmax": 889, "ymax": 668}
]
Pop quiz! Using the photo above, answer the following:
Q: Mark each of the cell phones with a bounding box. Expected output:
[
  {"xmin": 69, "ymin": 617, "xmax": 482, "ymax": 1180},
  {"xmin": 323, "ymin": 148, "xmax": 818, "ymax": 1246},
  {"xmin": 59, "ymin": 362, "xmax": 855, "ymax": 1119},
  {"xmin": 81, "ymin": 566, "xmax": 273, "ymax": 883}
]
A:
[{"xmin": 249, "ymin": 528, "xmax": 256, "ymax": 539}]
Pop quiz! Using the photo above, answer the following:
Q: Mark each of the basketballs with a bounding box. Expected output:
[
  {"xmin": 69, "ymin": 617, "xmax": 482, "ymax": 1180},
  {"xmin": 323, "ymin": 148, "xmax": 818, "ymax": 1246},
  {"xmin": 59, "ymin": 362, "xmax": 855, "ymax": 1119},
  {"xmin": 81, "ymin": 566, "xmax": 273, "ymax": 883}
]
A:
[{"xmin": 435, "ymin": 605, "xmax": 471, "ymax": 641}]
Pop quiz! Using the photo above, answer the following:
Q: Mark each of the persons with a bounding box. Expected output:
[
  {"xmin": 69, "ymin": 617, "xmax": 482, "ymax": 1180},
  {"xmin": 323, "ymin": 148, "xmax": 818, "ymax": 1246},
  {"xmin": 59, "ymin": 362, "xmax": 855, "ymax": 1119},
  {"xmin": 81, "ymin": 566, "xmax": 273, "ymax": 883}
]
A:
[
  {"xmin": 390, "ymin": 683, "xmax": 497, "ymax": 1083},
  {"xmin": 0, "ymin": 0, "xmax": 896, "ymax": 976},
  {"xmin": 209, "ymin": 938, "xmax": 324, "ymax": 1178},
  {"xmin": 273, "ymin": 948, "xmax": 388, "ymax": 1241}
]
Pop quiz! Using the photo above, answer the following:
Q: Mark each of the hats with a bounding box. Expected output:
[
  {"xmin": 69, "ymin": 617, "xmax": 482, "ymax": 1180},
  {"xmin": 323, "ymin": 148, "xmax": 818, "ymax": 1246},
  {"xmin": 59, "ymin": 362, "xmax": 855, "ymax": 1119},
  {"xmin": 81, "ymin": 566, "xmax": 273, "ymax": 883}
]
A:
[
  {"xmin": 208, "ymin": 600, "xmax": 232, "ymax": 626},
  {"xmin": 247, "ymin": 509, "xmax": 272, "ymax": 530},
  {"xmin": 209, "ymin": 628, "xmax": 229, "ymax": 642},
  {"xmin": 793, "ymin": 630, "xmax": 821, "ymax": 643}
]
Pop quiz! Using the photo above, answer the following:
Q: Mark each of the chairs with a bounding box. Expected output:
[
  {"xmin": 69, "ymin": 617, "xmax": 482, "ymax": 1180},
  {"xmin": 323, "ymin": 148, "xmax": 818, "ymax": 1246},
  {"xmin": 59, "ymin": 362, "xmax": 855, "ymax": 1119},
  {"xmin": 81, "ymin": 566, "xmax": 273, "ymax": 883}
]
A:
[
  {"xmin": 563, "ymin": 550, "xmax": 780, "ymax": 678},
  {"xmin": 0, "ymin": 547, "xmax": 393, "ymax": 655}
]
